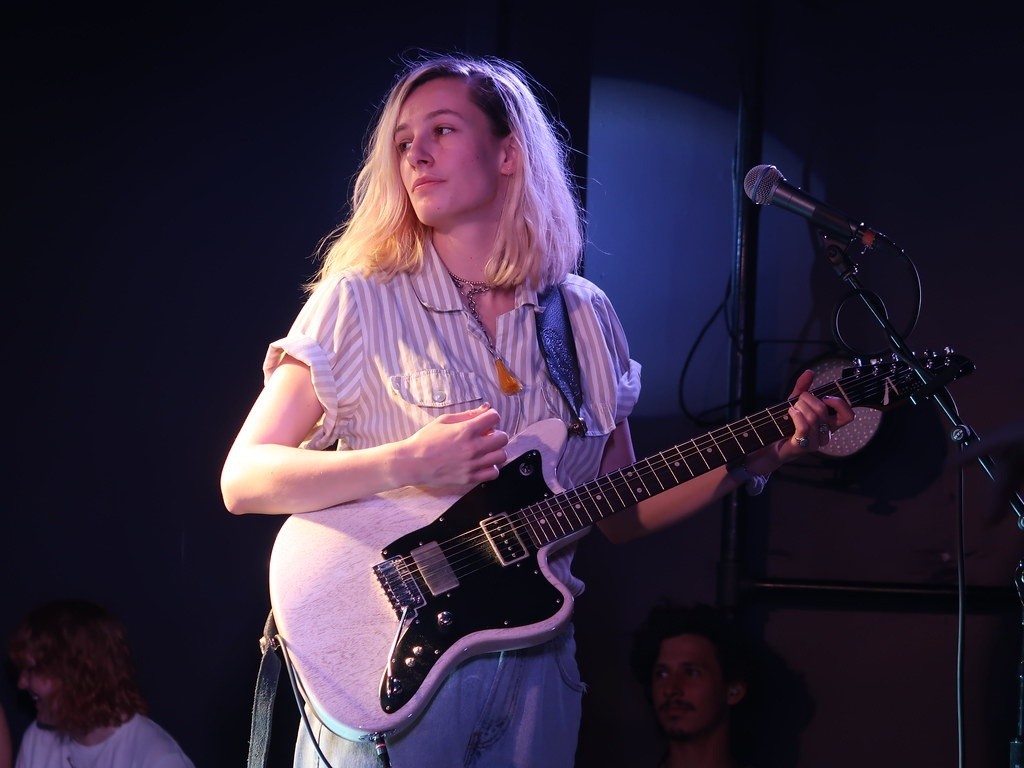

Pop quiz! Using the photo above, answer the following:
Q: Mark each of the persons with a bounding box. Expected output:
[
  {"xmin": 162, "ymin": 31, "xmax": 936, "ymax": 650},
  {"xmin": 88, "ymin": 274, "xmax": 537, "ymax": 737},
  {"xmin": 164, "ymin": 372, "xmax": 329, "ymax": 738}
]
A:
[
  {"xmin": 221, "ymin": 56, "xmax": 853, "ymax": 768},
  {"xmin": 629, "ymin": 596, "xmax": 760, "ymax": 768},
  {"xmin": 0, "ymin": 597, "xmax": 197, "ymax": 768}
]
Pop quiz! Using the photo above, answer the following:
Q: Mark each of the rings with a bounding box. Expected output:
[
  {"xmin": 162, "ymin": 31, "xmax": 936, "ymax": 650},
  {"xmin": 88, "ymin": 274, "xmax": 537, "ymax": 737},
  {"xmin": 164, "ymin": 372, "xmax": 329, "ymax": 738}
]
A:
[
  {"xmin": 820, "ymin": 424, "xmax": 829, "ymax": 434},
  {"xmin": 796, "ymin": 437, "xmax": 809, "ymax": 448}
]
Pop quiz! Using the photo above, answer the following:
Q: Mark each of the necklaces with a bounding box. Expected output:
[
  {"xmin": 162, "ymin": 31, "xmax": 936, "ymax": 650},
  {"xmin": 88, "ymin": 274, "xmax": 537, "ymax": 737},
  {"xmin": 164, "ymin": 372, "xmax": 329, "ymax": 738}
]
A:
[{"xmin": 447, "ymin": 271, "xmax": 523, "ymax": 395}]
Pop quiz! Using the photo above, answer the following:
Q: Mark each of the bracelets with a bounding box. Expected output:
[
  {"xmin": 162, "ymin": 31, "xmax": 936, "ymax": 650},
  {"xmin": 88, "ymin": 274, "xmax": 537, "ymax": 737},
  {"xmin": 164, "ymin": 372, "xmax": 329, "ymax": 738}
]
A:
[{"xmin": 725, "ymin": 458, "xmax": 772, "ymax": 497}]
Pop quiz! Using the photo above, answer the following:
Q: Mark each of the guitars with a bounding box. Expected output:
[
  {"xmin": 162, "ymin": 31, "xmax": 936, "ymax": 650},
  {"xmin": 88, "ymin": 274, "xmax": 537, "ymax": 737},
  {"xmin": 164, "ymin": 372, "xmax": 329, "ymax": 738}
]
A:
[{"xmin": 268, "ymin": 342, "xmax": 981, "ymax": 745}]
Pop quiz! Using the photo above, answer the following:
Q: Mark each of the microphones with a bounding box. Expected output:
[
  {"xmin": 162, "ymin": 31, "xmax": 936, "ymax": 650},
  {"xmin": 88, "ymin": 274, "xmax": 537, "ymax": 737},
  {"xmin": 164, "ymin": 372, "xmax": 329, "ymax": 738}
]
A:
[{"xmin": 744, "ymin": 164, "xmax": 885, "ymax": 241}]
